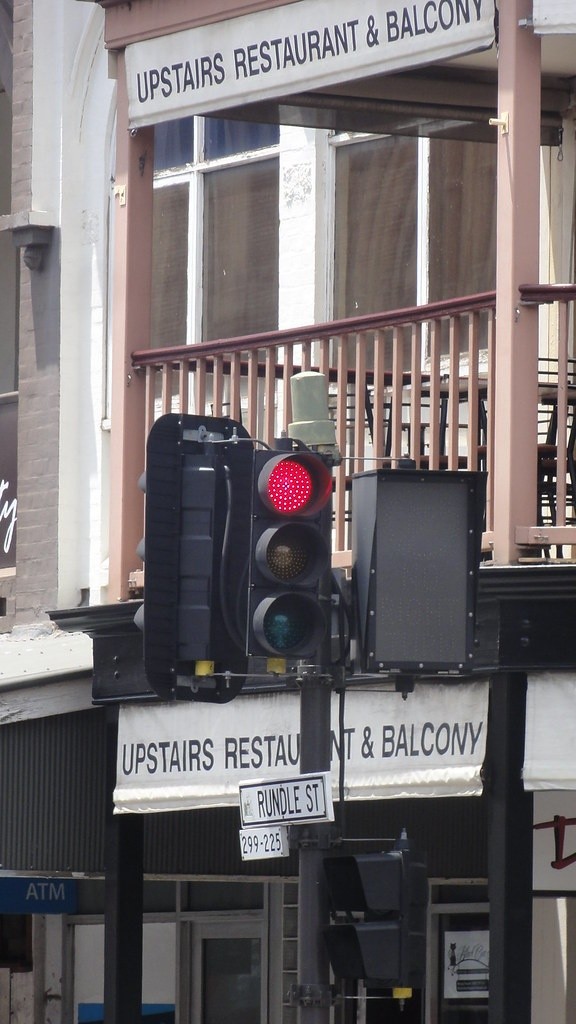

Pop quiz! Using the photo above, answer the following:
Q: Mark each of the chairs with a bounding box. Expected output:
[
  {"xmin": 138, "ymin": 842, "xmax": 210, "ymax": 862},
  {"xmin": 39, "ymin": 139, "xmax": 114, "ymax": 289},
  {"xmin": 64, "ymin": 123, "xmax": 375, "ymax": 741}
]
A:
[{"xmin": 364, "ymin": 375, "xmax": 576, "ymax": 558}]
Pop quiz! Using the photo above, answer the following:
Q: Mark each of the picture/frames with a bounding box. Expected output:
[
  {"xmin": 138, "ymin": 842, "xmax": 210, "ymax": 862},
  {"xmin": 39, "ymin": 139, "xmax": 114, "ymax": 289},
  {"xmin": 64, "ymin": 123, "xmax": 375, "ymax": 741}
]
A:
[{"xmin": 439, "ymin": 913, "xmax": 489, "ymax": 1004}]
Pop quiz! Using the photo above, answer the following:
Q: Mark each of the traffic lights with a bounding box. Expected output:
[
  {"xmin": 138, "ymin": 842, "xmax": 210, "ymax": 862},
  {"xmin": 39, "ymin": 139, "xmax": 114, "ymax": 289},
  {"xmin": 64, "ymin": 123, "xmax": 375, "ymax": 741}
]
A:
[
  {"xmin": 132, "ymin": 413, "xmax": 252, "ymax": 698},
  {"xmin": 351, "ymin": 468, "xmax": 490, "ymax": 674},
  {"xmin": 323, "ymin": 854, "xmax": 408, "ymax": 992},
  {"xmin": 246, "ymin": 447, "xmax": 334, "ymax": 659}
]
